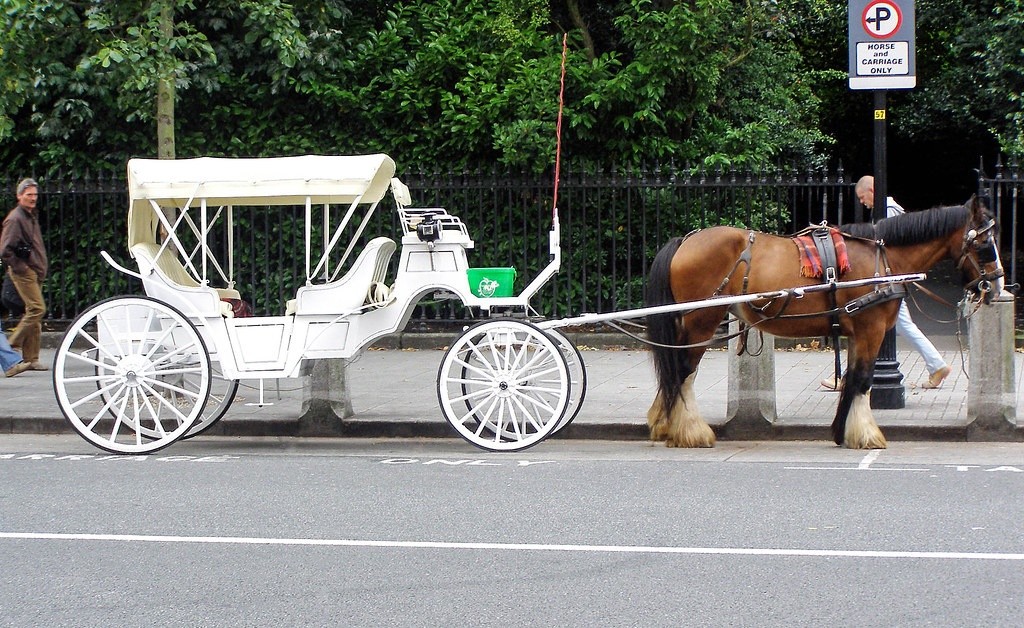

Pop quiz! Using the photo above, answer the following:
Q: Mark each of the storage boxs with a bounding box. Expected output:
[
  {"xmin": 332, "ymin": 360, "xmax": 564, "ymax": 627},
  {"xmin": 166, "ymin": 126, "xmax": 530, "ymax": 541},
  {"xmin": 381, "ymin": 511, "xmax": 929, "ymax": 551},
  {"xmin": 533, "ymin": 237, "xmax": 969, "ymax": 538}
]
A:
[{"xmin": 466, "ymin": 266, "xmax": 517, "ymax": 298}]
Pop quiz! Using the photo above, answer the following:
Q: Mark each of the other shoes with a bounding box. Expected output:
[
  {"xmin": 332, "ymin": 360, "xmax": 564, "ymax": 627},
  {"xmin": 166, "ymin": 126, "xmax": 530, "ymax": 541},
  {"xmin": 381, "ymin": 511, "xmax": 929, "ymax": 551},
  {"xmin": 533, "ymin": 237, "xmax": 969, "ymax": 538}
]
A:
[
  {"xmin": 921, "ymin": 366, "xmax": 954, "ymax": 390},
  {"xmin": 5, "ymin": 361, "xmax": 31, "ymax": 377},
  {"xmin": 822, "ymin": 374, "xmax": 843, "ymax": 390},
  {"xmin": 26, "ymin": 361, "xmax": 49, "ymax": 371}
]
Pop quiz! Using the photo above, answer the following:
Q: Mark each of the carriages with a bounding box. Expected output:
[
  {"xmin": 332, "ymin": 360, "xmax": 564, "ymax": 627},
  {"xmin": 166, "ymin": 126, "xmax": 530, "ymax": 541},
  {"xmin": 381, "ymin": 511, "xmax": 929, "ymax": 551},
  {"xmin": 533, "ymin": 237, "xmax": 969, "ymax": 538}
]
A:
[{"xmin": 53, "ymin": 153, "xmax": 1005, "ymax": 455}]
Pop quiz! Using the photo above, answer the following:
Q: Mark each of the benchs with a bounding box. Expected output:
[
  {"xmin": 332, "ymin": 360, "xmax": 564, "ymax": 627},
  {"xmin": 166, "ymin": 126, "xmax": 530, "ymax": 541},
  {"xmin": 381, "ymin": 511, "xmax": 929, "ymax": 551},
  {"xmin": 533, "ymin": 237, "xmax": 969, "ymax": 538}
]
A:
[
  {"xmin": 294, "ymin": 236, "xmax": 397, "ymax": 315},
  {"xmin": 390, "ymin": 177, "xmax": 475, "ymax": 249},
  {"xmin": 130, "ymin": 241, "xmax": 241, "ymax": 318}
]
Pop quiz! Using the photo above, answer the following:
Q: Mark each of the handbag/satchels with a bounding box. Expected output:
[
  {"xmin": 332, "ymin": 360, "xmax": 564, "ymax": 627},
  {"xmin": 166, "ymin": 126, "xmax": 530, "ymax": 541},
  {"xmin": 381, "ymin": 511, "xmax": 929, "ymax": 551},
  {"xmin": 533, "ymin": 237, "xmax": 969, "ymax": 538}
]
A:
[{"xmin": 15, "ymin": 244, "xmax": 33, "ymax": 258}]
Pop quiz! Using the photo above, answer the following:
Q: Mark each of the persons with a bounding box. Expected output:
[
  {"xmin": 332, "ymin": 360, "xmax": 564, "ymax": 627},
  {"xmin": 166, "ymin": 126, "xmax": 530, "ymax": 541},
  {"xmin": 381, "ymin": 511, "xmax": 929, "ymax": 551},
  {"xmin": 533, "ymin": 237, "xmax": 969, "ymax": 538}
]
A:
[
  {"xmin": 0, "ymin": 177, "xmax": 50, "ymax": 371},
  {"xmin": 0, "ymin": 322, "xmax": 31, "ymax": 377},
  {"xmin": 820, "ymin": 176, "xmax": 951, "ymax": 389}
]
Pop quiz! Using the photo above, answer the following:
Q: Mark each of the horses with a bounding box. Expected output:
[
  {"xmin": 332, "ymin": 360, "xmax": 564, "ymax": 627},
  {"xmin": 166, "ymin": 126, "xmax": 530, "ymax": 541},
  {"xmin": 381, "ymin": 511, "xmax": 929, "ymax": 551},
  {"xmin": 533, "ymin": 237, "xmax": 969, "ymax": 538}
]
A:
[{"xmin": 644, "ymin": 194, "xmax": 1004, "ymax": 451}]
